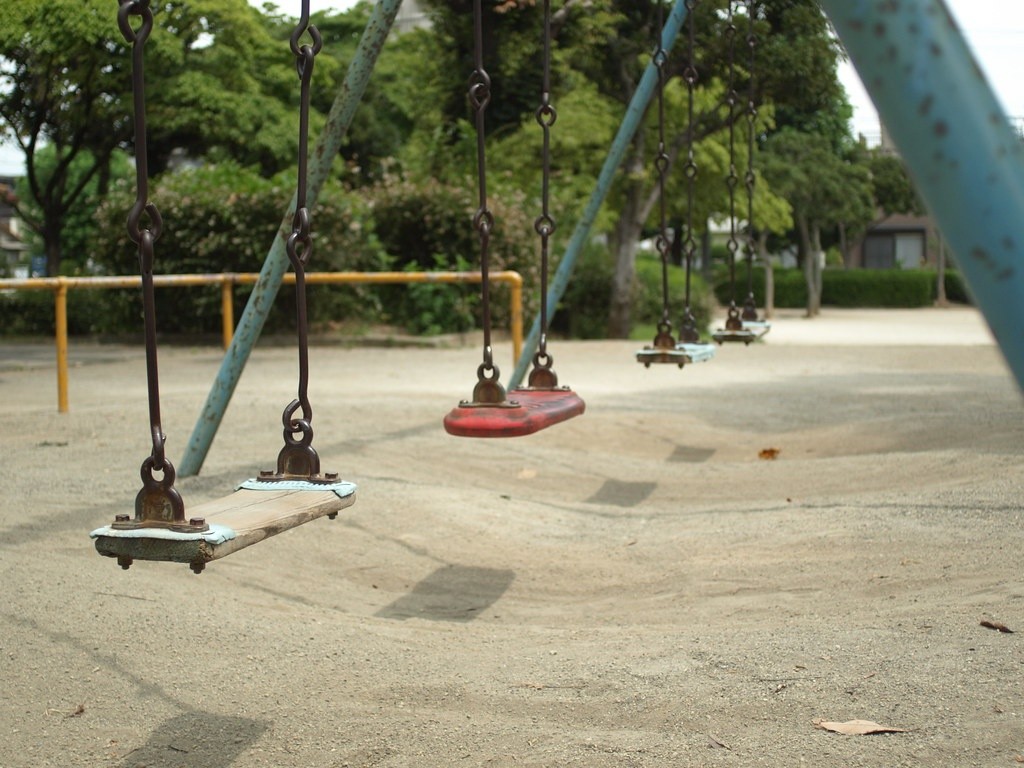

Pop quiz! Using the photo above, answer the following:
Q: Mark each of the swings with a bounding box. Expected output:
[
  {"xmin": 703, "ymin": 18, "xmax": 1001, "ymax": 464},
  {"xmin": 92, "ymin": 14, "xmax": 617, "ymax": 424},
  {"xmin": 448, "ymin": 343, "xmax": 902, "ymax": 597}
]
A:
[
  {"xmin": 444, "ymin": 0, "xmax": 586, "ymax": 437},
  {"xmin": 713, "ymin": 0, "xmax": 770, "ymax": 345},
  {"xmin": 636, "ymin": 0, "xmax": 716, "ymax": 369},
  {"xmin": 91, "ymin": 0, "xmax": 355, "ymax": 573}
]
